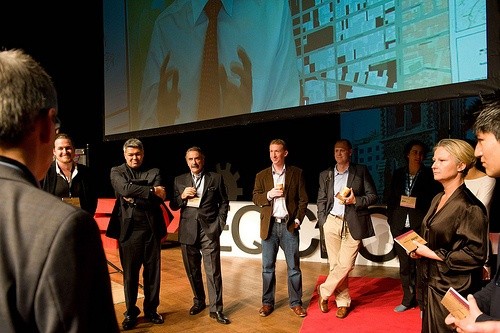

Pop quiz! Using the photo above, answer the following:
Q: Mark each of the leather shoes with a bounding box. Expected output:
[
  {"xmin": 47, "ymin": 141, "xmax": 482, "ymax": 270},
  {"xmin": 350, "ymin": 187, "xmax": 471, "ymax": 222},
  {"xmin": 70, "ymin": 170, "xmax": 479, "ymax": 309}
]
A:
[
  {"xmin": 290, "ymin": 305, "xmax": 306, "ymax": 317},
  {"xmin": 122, "ymin": 314, "xmax": 137, "ymax": 330},
  {"xmin": 317, "ymin": 285, "xmax": 328, "ymax": 313},
  {"xmin": 209, "ymin": 311, "xmax": 230, "ymax": 324},
  {"xmin": 144, "ymin": 313, "xmax": 163, "ymax": 324},
  {"xmin": 259, "ymin": 305, "xmax": 274, "ymax": 317},
  {"xmin": 189, "ymin": 303, "xmax": 206, "ymax": 315},
  {"xmin": 336, "ymin": 307, "xmax": 348, "ymax": 318}
]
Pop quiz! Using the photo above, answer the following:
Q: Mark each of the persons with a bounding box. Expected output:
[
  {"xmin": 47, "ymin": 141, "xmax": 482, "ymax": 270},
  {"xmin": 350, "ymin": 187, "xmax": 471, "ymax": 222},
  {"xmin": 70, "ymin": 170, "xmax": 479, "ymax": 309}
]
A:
[
  {"xmin": 169, "ymin": 147, "xmax": 230, "ymax": 324},
  {"xmin": 445, "ymin": 100, "xmax": 500, "ymax": 333},
  {"xmin": 411, "ymin": 138, "xmax": 487, "ymax": 333},
  {"xmin": 459, "ymin": 138, "xmax": 496, "ymax": 217},
  {"xmin": 138, "ymin": 0, "xmax": 300, "ymax": 130},
  {"xmin": 386, "ymin": 141, "xmax": 442, "ymax": 320},
  {"xmin": 314, "ymin": 139, "xmax": 379, "ymax": 319},
  {"xmin": 105, "ymin": 137, "xmax": 167, "ymax": 330},
  {"xmin": 252, "ymin": 139, "xmax": 308, "ymax": 317},
  {"xmin": 39, "ymin": 133, "xmax": 98, "ymax": 218},
  {"xmin": 0, "ymin": 48, "xmax": 120, "ymax": 333}
]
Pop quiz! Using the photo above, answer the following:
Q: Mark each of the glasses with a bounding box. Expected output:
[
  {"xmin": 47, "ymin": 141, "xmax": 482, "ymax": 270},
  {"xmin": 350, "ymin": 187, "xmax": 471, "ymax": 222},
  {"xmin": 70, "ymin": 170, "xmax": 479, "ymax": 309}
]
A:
[{"xmin": 54, "ymin": 114, "xmax": 61, "ymax": 130}]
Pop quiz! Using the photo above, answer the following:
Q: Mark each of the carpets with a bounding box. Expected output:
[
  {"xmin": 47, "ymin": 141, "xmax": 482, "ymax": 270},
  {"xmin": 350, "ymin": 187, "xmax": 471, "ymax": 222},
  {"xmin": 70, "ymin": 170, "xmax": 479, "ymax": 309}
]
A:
[
  {"xmin": 299, "ymin": 275, "xmax": 423, "ymax": 333},
  {"xmin": 111, "ymin": 281, "xmax": 145, "ymax": 305}
]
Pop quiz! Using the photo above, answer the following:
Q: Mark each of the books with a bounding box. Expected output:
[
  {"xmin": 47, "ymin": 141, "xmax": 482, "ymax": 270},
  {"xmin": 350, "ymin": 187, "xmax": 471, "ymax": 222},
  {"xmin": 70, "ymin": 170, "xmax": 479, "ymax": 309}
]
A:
[
  {"xmin": 394, "ymin": 230, "xmax": 427, "ymax": 253},
  {"xmin": 440, "ymin": 287, "xmax": 470, "ymax": 319}
]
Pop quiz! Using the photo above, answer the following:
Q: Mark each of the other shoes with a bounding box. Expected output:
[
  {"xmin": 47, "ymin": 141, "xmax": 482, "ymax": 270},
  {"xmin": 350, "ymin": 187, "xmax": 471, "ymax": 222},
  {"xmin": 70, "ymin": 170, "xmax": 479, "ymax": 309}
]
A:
[{"xmin": 394, "ymin": 304, "xmax": 407, "ymax": 312}]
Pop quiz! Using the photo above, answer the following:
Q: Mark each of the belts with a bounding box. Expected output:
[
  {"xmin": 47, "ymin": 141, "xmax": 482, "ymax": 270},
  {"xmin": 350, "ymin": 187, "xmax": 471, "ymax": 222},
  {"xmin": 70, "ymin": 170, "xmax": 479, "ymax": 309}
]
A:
[{"xmin": 271, "ymin": 218, "xmax": 288, "ymax": 223}]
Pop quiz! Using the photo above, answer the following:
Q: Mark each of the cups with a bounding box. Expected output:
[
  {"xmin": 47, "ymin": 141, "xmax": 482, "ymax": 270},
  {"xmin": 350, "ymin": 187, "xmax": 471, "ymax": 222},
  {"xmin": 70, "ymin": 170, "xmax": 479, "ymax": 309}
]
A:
[
  {"xmin": 276, "ymin": 183, "xmax": 283, "ymax": 191},
  {"xmin": 190, "ymin": 187, "xmax": 195, "ymax": 196},
  {"xmin": 339, "ymin": 186, "xmax": 351, "ymax": 196}
]
